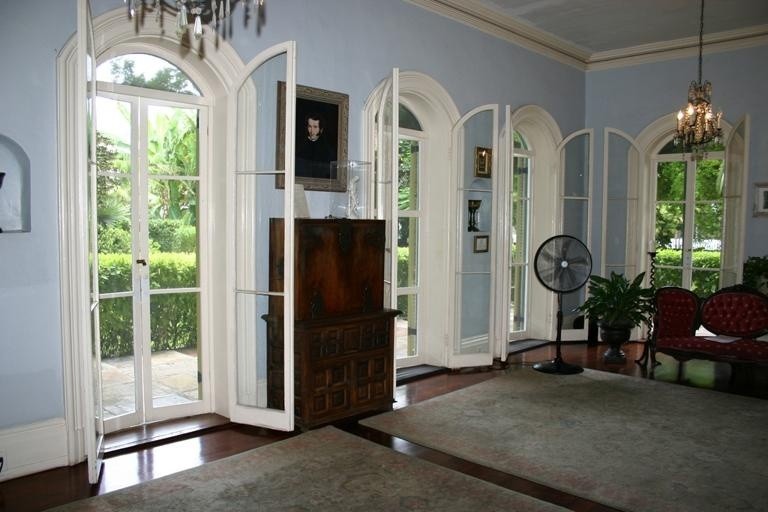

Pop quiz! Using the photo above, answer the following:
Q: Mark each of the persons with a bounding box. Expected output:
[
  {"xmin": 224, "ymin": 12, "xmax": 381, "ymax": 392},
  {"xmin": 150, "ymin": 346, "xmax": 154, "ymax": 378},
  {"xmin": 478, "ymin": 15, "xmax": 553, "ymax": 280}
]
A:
[{"xmin": 295, "ymin": 112, "xmax": 333, "ymax": 178}]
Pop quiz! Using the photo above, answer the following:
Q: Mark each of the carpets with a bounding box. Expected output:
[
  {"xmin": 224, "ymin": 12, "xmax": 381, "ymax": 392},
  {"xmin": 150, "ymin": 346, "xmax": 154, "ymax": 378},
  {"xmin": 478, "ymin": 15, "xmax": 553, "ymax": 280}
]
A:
[
  {"xmin": 43, "ymin": 425, "xmax": 575, "ymax": 512},
  {"xmin": 358, "ymin": 367, "xmax": 768, "ymax": 512}
]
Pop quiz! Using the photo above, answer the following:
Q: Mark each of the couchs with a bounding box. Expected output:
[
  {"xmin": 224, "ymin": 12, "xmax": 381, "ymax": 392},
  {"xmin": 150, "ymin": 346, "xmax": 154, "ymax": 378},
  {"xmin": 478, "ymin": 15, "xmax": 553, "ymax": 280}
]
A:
[{"xmin": 635, "ymin": 284, "xmax": 767, "ymax": 391}]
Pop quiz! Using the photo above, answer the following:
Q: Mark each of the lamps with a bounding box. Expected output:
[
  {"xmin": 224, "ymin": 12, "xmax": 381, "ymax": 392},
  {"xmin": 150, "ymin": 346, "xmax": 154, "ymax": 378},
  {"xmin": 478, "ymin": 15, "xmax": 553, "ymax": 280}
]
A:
[
  {"xmin": 674, "ymin": 0, "xmax": 723, "ymax": 146},
  {"xmin": 124, "ymin": 0, "xmax": 264, "ymax": 40},
  {"xmin": 468, "ymin": 200, "xmax": 482, "ymax": 232}
]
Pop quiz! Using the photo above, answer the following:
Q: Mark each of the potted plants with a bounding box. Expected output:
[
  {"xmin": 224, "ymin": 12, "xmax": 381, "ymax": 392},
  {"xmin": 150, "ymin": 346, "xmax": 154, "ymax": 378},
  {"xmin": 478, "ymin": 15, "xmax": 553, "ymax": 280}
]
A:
[{"xmin": 572, "ymin": 271, "xmax": 655, "ymax": 364}]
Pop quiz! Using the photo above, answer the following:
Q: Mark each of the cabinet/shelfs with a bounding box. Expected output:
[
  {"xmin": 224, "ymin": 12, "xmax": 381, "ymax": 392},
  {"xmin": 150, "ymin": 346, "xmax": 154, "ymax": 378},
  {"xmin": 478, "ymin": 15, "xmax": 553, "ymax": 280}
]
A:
[{"xmin": 260, "ymin": 218, "xmax": 403, "ymax": 432}]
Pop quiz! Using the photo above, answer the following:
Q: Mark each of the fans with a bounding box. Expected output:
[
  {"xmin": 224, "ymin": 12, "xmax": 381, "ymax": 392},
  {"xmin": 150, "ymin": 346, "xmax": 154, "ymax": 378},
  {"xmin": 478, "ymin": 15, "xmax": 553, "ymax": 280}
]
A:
[{"xmin": 532, "ymin": 235, "xmax": 592, "ymax": 374}]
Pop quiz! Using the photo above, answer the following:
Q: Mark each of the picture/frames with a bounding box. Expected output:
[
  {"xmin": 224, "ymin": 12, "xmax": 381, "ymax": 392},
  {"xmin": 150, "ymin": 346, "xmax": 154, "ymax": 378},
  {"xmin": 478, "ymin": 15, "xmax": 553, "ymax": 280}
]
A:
[
  {"xmin": 474, "ymin": 145, "xmax": 492, "ymax": 178},
  {"xmin": 752, "ymin": 181, "xmax": 768, "ymax": 218},
  {"xmin": 275, "ymin": 80, "xmax": 349, "ymax": 192},
  {"xmin": 473, "ymin": 235, "xmax": 489, "ymax": 253}
]
